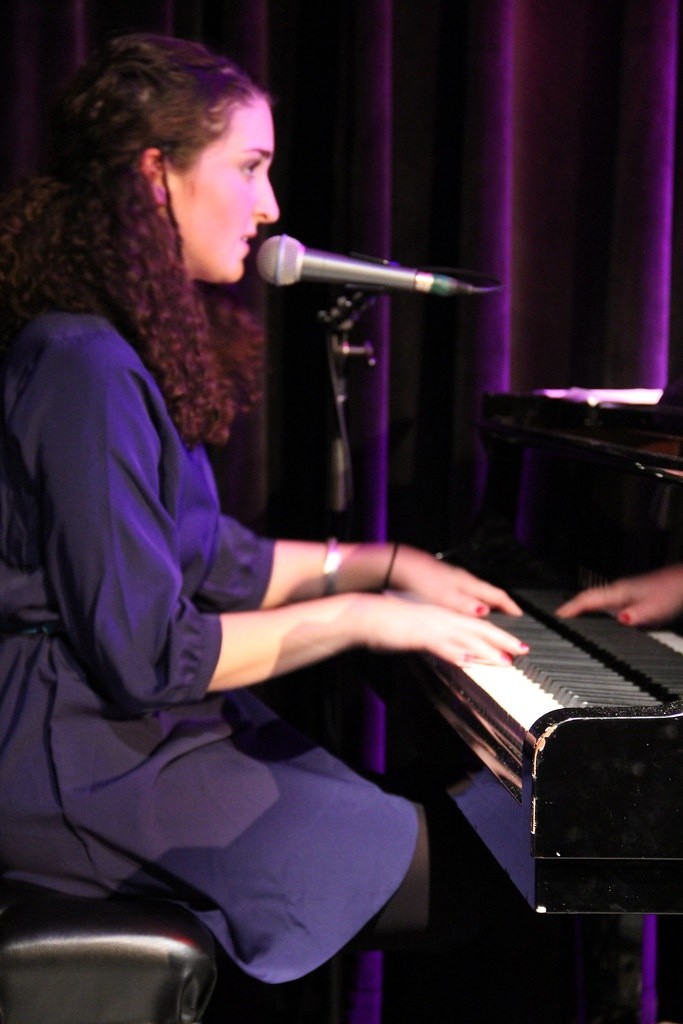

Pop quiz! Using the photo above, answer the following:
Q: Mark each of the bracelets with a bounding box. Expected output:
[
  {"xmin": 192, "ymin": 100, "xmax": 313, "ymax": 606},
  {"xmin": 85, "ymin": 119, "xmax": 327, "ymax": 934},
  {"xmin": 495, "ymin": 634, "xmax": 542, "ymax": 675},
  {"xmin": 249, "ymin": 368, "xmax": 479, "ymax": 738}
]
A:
[
  {"xmin": 323, "ymin": 537, "xmax": 338, "ymax": 597},
  {"xmin": 381, "ymin": 543, "xmax": 398, "ymax": 591}
]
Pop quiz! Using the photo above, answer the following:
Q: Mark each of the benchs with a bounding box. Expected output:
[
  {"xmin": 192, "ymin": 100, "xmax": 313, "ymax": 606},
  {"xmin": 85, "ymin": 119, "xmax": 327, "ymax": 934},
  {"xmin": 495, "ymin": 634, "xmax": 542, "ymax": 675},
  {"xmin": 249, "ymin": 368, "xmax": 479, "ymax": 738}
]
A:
[{"xmin": 0, "ymin": 899, "xmax": 215, "ymax": 1023}]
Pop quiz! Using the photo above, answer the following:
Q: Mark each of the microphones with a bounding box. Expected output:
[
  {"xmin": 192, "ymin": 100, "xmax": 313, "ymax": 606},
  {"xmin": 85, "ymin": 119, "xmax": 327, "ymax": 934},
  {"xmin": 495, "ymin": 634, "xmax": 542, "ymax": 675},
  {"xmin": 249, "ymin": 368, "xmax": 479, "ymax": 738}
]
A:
[{"xmin": 254, "ymin": 234, "xmax": 475, "ymax": 298}]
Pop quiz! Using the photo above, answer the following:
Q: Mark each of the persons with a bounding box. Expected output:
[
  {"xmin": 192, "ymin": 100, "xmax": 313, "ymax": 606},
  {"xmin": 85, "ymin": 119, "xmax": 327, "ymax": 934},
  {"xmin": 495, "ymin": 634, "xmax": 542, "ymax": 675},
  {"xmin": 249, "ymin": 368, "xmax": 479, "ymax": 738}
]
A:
[
  {"xmin": 556, "ymin": 567, "xmax": 683, "ymax": 632},
  {"xmin": 0, "ymin": 33, "xmax": 529, "ymax": 1024}
]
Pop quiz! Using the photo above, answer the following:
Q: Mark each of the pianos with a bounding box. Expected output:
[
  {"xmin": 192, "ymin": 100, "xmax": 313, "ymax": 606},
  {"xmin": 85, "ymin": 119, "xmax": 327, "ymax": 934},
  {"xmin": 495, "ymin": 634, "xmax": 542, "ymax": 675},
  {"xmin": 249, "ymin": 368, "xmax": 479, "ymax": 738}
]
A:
[{"xmin": 371, "ymin": 377, "xmax": 683, "ymax": 1024}]
